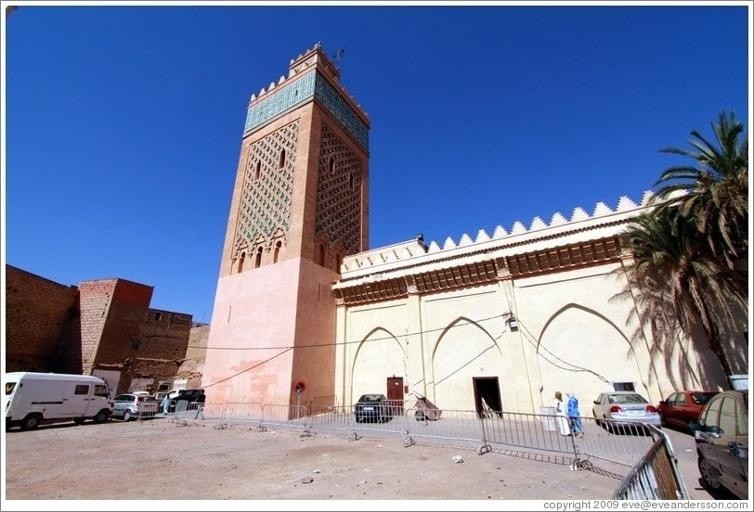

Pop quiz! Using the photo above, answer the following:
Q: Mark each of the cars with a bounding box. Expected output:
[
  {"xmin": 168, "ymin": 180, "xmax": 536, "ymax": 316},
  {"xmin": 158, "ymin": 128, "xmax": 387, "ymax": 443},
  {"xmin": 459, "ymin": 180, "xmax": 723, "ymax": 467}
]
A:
[
  {"xmin": 689, "ymin": 390, "xmax": 748, "ymax": 499},
  {"xmin": 657, "ymin": 392, "xmax": 720, "ymax": 435},
  {"xmin": 356, "ymin": 394, "xmax": 391, "ymax": 423},
  {"xmin": 593, "ymin": 392, "xmax": 662, "ymax": 431}
]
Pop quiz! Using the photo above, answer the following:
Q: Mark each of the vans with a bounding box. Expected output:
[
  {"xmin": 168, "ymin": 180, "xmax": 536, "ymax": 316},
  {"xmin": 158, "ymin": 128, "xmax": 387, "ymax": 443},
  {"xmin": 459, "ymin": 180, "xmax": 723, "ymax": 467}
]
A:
[
  {"xmin": 133, "ymin": 388, "xmax": 201, "ymax": 411},
  {"xmin": 6, "ymin": 371, "xmax": 114, "ymax": 431}
]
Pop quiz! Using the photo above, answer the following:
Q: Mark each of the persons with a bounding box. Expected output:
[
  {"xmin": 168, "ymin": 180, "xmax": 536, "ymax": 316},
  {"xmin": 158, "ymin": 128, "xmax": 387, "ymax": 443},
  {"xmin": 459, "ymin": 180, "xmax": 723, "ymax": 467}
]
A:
[
  {"xmin": 566, "ymin": 391, "xmax": 584, "ymax": 438},
  {"xmin": 160, "ymin": 392, "xmax": 171, "ymax": 416},
  {"xmin": 553, "ymin": 391, "xmax": 571, "ymax": 436},
  {"xmin": 194, "ymin": 389, "xmax": 206, "ymax": 420}
]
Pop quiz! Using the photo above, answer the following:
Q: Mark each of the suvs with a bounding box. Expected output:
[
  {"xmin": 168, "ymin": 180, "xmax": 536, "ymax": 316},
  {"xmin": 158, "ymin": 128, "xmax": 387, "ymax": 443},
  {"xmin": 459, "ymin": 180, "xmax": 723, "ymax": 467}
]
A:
[{"xmin": 108, "ymin": 394, "xmax": 159, "ymax": 421}]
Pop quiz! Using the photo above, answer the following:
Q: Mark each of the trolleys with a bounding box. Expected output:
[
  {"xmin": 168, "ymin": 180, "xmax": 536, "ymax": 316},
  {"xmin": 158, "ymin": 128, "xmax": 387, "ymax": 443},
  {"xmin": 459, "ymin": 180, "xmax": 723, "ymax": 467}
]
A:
[{"xmin": 406, "ymin": 390, "xmax": 442, "ymax": 421}]
